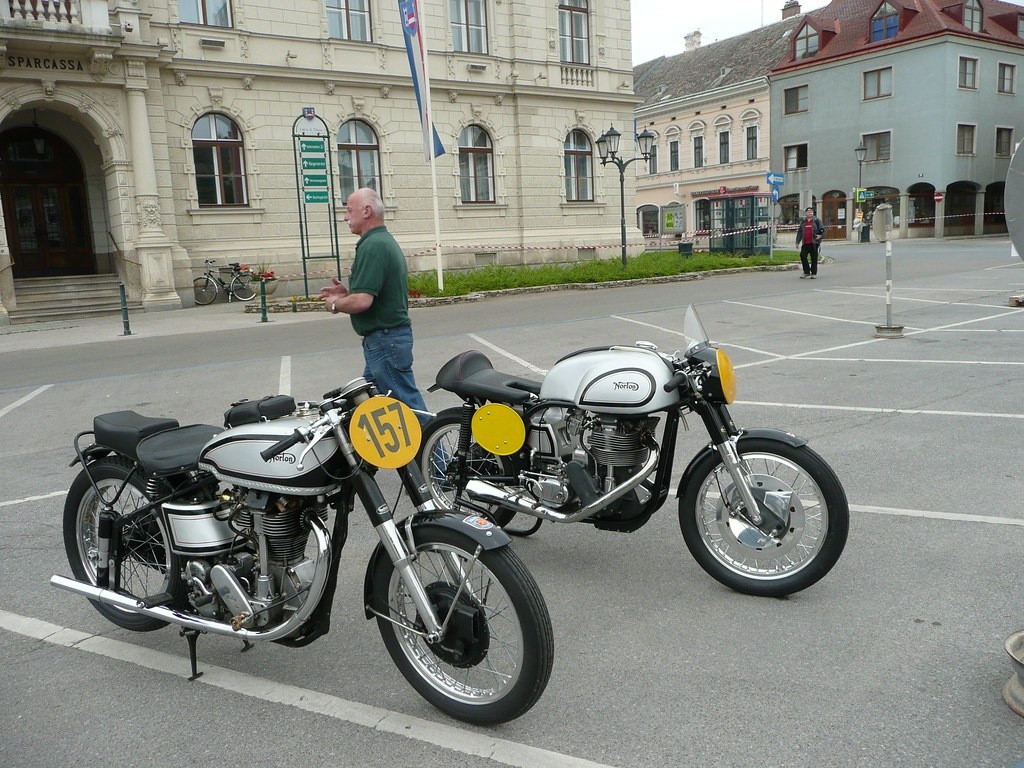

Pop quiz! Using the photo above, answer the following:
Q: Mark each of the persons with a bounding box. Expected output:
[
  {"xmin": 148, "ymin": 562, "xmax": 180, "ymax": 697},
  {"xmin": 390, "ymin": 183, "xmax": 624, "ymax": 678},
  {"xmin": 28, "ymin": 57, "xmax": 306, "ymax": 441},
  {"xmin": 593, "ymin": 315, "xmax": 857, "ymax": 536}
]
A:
[
  {"xmin": 795, "ymin": 206, "xmax": 826, "ymax": 281},
  {"xmin": 314, "ymin": 189, "xmax": 462, "ymax": 493}
]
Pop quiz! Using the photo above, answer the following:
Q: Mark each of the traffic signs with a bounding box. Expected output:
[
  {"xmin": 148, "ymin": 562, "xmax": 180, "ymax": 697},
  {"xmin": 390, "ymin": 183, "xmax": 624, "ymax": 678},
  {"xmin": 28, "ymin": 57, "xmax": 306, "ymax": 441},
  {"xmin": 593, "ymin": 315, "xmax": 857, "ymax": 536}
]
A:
[
  {"xmin": 303, "ymin": 174, "xmax": 327, "ymax": 186},
  {"xmin": 305, "ymin": 190, "xmax": 329, "ymax": 203},
  {"xmin": 300, "ymin": 140, "xmax": 326, "ymax": 153},
  {"xmin": 301, "ymin": 158, "xmax": 326, "ymax": 169}
]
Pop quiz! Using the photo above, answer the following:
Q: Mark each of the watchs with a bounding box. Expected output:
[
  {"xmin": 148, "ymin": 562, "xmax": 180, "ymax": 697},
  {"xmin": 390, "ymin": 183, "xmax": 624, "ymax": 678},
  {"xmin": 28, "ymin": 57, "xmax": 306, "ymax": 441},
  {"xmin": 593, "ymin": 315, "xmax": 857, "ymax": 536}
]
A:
[{"xmin": 332, "ymin": 299, "xmax": 338, "ymax": 312}]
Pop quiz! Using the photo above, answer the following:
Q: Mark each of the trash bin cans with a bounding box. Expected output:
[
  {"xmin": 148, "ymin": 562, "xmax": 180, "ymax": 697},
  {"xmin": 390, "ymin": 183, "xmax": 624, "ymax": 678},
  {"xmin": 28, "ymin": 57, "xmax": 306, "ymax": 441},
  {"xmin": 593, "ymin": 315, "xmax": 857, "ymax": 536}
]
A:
[
  {"xmin": 860, "ymin": 224, "xmax": 870, "ymax": 244},
  {"xmin": 678, "ymin": 243, "xmax": 692, "ymax": 260}
]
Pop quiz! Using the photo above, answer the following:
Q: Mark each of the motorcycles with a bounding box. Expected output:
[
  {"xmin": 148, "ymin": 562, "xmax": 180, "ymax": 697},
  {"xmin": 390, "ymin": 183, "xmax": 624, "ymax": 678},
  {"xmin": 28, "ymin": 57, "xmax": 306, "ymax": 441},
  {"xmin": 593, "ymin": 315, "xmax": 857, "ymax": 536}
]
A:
[
  {"xmin": 50, "ymin": 376, "xmax": 557, "ymax": 728},
  {"xmin": 416, "ymin": 300, "xmax": 850, "ymax": 600}
]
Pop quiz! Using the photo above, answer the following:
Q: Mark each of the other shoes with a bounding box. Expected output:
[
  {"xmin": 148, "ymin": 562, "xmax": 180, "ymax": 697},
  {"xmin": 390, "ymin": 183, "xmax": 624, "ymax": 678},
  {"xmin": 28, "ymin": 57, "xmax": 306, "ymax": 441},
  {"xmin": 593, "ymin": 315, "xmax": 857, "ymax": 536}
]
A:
[
  {"xmin": 800, "ymin": 273, "xmax": 811, "ymax": 279},
  {"xmin": 811, "ymin": 274, "xmax": 817, "ymax": 279},
  {"xmin": 405, "ymin": 480, "xmax": 455, "ymax": 496}
]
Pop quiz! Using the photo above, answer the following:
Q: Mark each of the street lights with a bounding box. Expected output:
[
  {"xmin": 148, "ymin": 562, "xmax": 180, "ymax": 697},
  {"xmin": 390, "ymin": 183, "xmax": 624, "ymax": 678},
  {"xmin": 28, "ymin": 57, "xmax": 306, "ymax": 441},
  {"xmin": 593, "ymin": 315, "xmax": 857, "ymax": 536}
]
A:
[
  {"xmin": 595, "ymin": 123, "xmax": 655, "ymax": 268},
  {"xmin": 855, "ymin": 140, "xmax": 867, "ymax": 210}
]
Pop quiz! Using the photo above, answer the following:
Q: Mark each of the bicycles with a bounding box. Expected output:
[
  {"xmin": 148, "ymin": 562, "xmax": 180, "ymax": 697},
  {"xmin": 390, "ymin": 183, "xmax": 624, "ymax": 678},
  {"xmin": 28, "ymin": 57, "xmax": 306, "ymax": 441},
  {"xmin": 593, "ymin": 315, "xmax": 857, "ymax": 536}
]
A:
[{"xmin": 193, "ymin": 259, "xmax": 258, "ymax": 305}]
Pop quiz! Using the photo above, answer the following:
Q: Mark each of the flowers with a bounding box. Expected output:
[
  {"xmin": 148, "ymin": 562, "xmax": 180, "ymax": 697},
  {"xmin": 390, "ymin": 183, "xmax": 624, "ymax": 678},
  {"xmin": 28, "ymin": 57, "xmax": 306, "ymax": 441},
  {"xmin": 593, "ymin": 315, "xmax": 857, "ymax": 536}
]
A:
[{"xmin": 248, "ymin": 256, "xmax": 276, "ymax": 281}]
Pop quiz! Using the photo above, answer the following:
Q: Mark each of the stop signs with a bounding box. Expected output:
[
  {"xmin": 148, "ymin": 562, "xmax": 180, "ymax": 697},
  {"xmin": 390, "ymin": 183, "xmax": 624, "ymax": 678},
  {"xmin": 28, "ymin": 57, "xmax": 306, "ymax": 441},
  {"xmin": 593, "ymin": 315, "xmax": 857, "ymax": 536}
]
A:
[{"xmin": 933, "ymin": 193, "xmax": 944, "ymax": 203}]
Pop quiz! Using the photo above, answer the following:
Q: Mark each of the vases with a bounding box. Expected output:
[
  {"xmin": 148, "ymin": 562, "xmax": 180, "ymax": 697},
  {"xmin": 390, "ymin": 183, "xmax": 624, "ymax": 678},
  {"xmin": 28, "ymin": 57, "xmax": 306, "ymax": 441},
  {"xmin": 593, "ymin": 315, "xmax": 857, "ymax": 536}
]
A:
[{"xmin": 250, "ymin": 280, "xmax": 278, "ymax": 295}]
